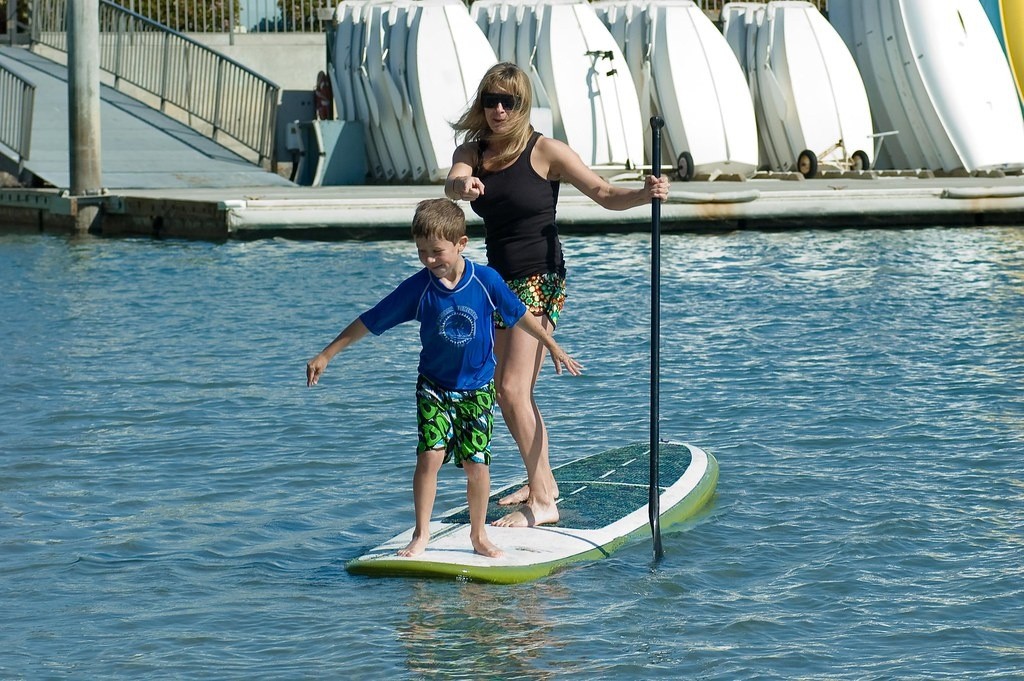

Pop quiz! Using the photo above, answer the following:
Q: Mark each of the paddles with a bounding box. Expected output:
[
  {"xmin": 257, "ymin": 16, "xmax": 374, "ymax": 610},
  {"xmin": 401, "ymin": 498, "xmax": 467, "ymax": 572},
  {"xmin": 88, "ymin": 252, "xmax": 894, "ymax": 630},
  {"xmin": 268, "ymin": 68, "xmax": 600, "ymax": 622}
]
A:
[{"xmin": 650, "ymin": 113, "xmax": 664, "ymax": 566}]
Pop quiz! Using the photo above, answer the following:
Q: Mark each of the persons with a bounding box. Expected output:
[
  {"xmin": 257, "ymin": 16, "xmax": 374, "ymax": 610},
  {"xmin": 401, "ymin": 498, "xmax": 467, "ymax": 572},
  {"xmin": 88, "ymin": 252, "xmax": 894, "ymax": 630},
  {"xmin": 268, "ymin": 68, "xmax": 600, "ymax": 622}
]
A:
[
  {"xmin": 305, "ymin": 196, "xmax": 584, "ymax": 558},
  {"xmin": 445, "ymin": 61, "xmax": 672, "ymax": 528}
]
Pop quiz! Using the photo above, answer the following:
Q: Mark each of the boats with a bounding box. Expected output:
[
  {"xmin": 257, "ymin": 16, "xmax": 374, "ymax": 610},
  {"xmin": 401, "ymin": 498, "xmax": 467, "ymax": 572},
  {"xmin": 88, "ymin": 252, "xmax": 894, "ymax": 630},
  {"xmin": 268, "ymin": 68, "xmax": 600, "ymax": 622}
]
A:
[
  {"xmin": 590, "ymin": 0, "xmax": 759, "ymax": 181},
  {"xmin": 470, "ymin": 0, "xmax": 645, "ymax": 183},
  {"xmin": 326, "ymin": 0, "xmax": 500, "ymax": 186},
  {"xmin": 825, "ymin": 0, "xmax": 1024, "ymax": 173},
  {"xmin": 721, "ymin": 0, "xmax": 874, "ymax": 172}
]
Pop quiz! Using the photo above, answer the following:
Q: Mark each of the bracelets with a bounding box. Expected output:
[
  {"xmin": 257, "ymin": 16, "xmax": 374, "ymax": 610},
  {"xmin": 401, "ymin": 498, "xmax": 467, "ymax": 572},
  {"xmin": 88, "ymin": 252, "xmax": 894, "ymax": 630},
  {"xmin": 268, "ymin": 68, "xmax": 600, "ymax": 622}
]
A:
[{"xmin": 452, "ymin": 179, "xmax": 460, "ymax": 194}]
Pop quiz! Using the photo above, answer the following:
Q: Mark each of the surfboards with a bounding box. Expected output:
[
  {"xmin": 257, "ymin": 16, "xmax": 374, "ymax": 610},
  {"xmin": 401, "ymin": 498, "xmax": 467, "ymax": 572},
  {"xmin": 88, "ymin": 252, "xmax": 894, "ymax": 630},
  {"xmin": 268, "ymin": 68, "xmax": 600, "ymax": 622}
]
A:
[{"xmin": 346, "ymin": 438, "xmax": 720, "ymax": 586}]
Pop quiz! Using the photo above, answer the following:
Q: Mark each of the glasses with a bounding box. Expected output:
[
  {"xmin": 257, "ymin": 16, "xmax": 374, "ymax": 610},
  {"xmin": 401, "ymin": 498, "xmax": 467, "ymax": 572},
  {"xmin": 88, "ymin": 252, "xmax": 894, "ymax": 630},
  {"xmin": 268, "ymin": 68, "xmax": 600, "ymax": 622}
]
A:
[{"xmin": 480, "ymin": 92, "xmax": 523, "ymax": 110}]
